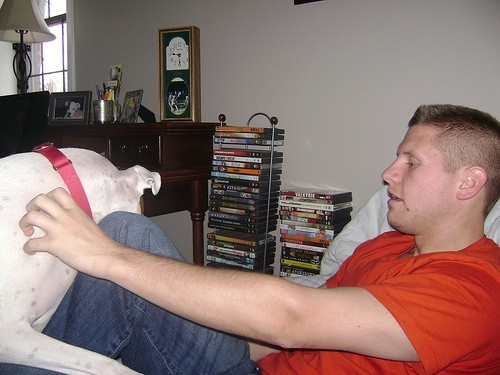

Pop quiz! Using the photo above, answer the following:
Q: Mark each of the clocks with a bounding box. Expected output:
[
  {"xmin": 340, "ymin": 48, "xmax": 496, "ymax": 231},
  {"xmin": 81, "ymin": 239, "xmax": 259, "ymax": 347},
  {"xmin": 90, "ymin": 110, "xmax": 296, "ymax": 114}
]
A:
[{"xmin": 158, "ymin": 26, "xmax": 201, "ymax": 123}]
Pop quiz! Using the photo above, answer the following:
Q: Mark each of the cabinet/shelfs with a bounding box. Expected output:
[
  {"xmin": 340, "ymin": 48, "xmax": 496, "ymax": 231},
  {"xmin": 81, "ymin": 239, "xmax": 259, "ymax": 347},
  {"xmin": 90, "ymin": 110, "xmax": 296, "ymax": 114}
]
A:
[{"xmin": 48, "ymin": 123, "xmax": 226, "ymax": 267}]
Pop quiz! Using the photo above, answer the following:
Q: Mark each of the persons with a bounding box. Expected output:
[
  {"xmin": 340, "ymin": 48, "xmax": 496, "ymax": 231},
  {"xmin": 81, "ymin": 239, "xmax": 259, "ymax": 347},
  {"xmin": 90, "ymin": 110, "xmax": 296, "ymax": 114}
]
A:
[{"xmin": 0, "ymin": 104, "xmax": 500, "ymax": 375}]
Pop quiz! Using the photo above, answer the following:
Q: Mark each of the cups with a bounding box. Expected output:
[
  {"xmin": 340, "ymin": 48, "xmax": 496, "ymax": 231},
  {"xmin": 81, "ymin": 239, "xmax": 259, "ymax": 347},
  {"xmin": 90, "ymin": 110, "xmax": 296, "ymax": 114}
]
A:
[{"xmin": 93, "ymin": 99, "xmax": 114, "ymax": 124}]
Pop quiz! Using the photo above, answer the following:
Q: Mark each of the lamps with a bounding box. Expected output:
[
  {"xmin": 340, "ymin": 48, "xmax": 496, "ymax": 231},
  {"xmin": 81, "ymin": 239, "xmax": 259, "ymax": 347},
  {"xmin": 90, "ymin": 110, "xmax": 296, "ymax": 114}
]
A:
[{"xmin": 0, "ymin": 0, "xmax": 56, "ymax": 95}]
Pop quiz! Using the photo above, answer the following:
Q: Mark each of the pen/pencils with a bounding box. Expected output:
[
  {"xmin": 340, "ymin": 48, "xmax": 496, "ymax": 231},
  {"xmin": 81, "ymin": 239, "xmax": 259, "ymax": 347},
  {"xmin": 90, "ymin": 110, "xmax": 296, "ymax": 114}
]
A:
[{"xmin": 95, "ymin": 82, "xmax": 115, "ymax": 100}]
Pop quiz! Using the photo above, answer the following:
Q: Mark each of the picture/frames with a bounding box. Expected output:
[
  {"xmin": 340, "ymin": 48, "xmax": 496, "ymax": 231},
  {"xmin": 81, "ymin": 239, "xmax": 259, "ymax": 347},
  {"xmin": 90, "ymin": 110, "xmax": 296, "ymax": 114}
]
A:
[
  {"xmin": 119, "ymin": 90, "xmax": 144, "ymax": 123},
  {"xmin": 49, "ymin": 91, "xmax": 92, "ymax": 125}
]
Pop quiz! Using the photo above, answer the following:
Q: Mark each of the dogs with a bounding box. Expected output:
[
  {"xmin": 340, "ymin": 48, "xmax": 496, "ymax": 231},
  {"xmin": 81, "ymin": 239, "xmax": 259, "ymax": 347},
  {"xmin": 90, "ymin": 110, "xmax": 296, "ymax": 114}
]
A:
[{"xmin": 0, "ymin": 142, "xmax": 162, "ymax": 375}]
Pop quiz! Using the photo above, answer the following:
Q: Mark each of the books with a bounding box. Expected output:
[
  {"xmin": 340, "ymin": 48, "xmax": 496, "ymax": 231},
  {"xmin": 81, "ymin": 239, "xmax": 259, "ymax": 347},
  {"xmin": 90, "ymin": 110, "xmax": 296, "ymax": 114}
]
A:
[{"xmin": 206, "ymin": 125, "xmax": 352, "ymax": 278}]
